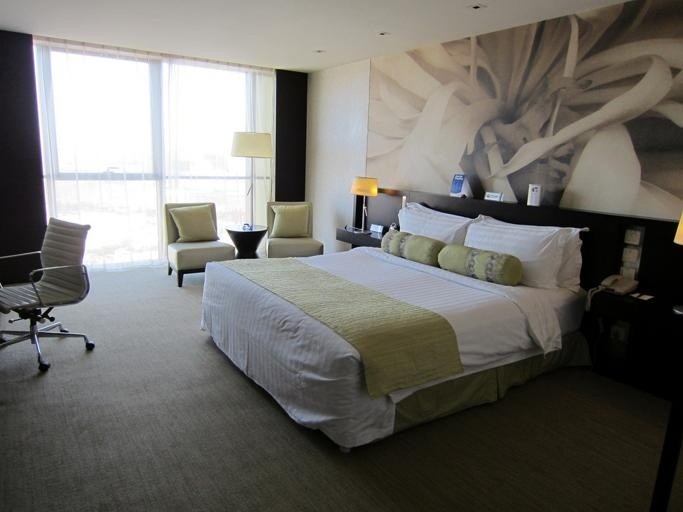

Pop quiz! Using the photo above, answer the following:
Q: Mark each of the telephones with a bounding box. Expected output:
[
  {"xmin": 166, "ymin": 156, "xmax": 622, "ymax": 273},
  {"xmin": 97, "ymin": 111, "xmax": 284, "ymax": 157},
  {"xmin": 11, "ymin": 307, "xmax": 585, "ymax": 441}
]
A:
[{"xmin": 599, "ymin": 275, "xmax": 639, "ymax": 295}]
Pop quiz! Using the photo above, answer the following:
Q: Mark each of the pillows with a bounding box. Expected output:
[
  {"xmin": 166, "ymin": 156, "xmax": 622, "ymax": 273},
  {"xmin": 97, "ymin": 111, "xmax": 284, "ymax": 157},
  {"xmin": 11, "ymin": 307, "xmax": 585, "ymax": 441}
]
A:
[
  {"xmin": 169, "ymin": 203, "xmax": 219, "ymax": 244},
  {"xmin": 380, "ymin": 201, "xmax": 590, "ymax": 294},
  {"xmin": 269, "ymin": 203, "xmax": 309, "ymax": 238}
]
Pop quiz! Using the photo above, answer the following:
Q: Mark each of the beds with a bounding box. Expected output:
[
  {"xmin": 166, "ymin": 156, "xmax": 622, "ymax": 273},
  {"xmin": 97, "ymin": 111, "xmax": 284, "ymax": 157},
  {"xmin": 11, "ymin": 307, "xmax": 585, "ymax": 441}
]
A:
[{"xmin": 198, "ymin": 246, "xmax": 588, "ymax": 458}]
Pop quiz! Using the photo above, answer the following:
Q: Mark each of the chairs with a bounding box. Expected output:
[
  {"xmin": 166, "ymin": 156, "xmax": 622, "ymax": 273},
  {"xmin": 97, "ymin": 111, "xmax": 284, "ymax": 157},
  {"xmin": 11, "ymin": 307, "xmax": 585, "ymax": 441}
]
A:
[
  {"xmin": 0, "ymin": 216, "xmax": 96, "ymax": 375},
  {"xmin": 163, "ymin": 200, "xmax": 235, "ymax": 289},
  {"xmin": 264, "ymin": 201, "xmax": 324, "ymax": 258}
]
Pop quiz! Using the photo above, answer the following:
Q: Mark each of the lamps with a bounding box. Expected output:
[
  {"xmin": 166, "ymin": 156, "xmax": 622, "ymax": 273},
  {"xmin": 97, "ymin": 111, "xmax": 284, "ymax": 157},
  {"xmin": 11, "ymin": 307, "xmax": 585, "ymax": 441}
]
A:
[
  {"xmin": 350, "ymin": 176, "xmax": 378, "ymax": 235},
  {"xmin": 229, "ymin": 131, "xmax": 273, "ymax": 227}
]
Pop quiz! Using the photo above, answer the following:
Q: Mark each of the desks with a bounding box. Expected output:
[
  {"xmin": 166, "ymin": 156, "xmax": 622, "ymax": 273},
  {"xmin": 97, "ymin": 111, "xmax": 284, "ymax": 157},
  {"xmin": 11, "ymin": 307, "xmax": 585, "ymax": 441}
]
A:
[
  {"xmin": 226, "ymin": 225, "xmax": 268, "ymax": 259},
  {"xmin": 589, "ymin": 290, "xmax": 683, "ymax": 402},
  {"xmin": 336, "ymin": 226, "xmax": 384, "ymax": 247}
]
